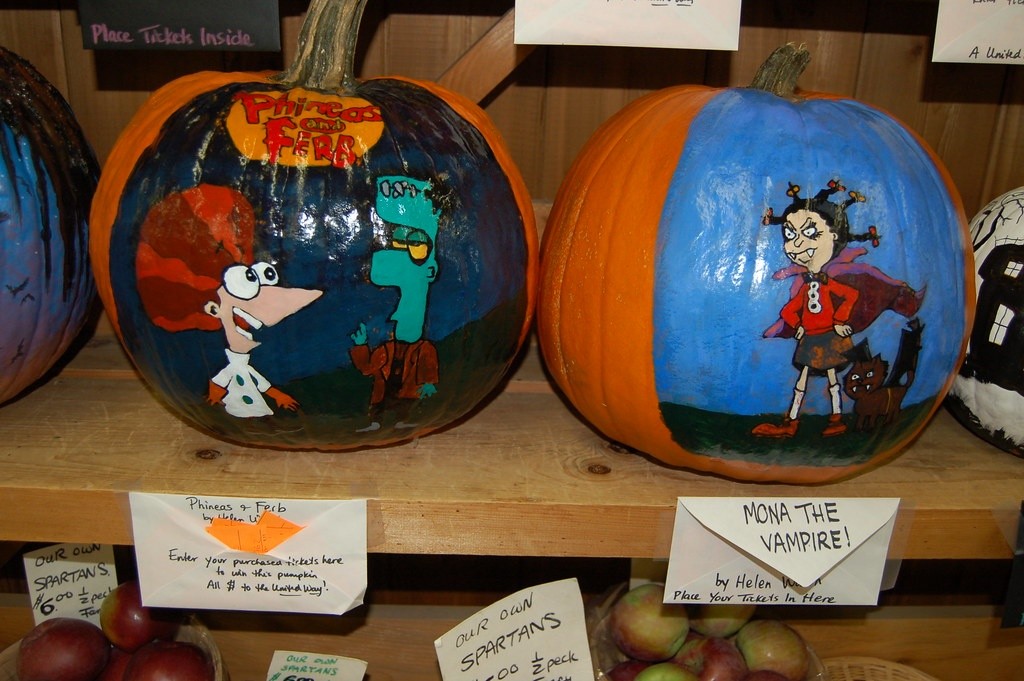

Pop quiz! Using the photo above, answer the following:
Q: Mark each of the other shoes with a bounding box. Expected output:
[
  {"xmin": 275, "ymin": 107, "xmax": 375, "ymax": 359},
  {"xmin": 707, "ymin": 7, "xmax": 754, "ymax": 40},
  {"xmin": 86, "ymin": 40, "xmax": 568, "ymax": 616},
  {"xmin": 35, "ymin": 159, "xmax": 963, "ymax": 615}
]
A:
[
  {"xmin": 821, "ymin": 412, "xmax": 847, "ymax": 438},
  {"xmin": 752, "ymin": 413, "xmax": 799, "ymax": 438}
]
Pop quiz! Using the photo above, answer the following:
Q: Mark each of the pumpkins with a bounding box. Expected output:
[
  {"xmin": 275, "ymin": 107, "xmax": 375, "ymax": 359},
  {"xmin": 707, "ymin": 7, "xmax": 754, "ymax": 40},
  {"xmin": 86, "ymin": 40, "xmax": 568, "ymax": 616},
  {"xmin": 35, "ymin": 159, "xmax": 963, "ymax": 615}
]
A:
[{"xmin": 0, "ymin": 1, "xmax": 1024, "ymax": 486}]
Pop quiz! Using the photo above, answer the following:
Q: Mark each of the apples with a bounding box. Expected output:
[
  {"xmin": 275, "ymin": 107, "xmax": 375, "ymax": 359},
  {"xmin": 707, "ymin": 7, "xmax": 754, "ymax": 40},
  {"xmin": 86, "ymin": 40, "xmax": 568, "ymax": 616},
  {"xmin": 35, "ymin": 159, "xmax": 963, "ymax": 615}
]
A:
[
  {"xmin": 16, "ymin": 581, "xmax": 217, "ymax": 681},
  {"xmin": 606, "ymin": 582, "xmax": 809, "ymax": 680}
]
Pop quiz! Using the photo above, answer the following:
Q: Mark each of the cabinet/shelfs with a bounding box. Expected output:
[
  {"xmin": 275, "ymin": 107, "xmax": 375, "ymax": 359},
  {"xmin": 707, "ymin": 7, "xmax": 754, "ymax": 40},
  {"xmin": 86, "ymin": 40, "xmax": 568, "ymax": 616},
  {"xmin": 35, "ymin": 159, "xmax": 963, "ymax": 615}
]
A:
[{"xmin": 0, "ymin": 1, "xmax": 1024, "ymax": 681}]
